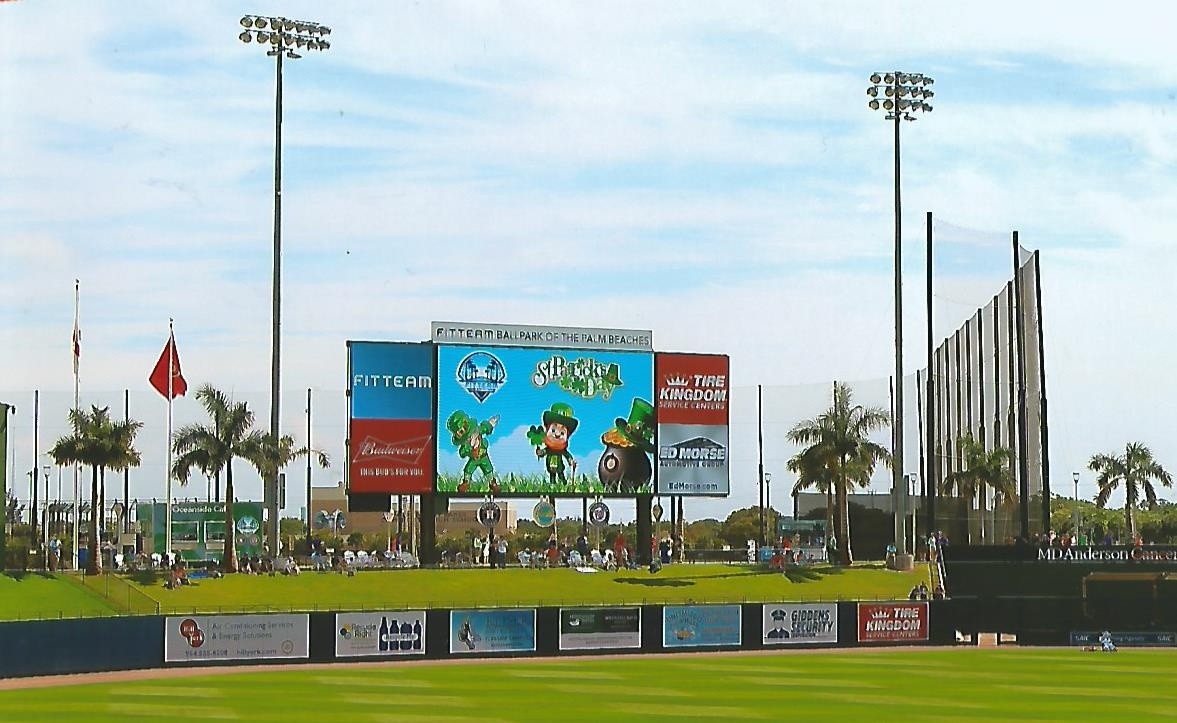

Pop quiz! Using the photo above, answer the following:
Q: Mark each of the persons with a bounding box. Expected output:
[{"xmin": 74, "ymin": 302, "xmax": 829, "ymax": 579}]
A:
[
  {"xmin": 1100, "ymin": 632, "xmax": 1119, "ymax": 650},
  {"xmin": 1049, "ymin": 530, "xmax": 1143, "ymax": 558},
  {"xmin": 163, "ymin": 554, "xmax": 357, "ymax": 589},
  {"xmin": 766, "ymin": 532, "xmax": 947, "ymax": 599},
  {"xmin": 474, "ymin": 533, "xmax": 685, "ymax": 572},
  {"xmin": 50, "ymin": 534, "xmax": 62, "ymax": 571}
]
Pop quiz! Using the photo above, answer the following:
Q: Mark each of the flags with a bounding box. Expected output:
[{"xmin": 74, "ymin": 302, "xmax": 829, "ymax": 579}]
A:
[{"xmin": 149, "ymin": 331, "xmax": 187, "ymax": 400}]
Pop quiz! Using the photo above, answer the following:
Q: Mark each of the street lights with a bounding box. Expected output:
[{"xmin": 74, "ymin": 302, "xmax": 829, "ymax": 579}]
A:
[
  {"xmin": 764, "ymin": 473, "xmax": 771, "ymax": 509},
  {"xmin": 1073, "ymin": 472, "xmax": 1080, "ymax": 544},
  {"xmin": 236, "ymin": 11, "xmax": 331, "ymax": 571},
  {"xmin": 866, "ymin": 71, "xmax": 935, "ymax": 548},
  {"xmin": 909, "ymin": 472, "xmax": 917, "ymax": 557},
  {"xmin": 43, "ymin": 465, "xmax": 52, "ymax": 571}
]
